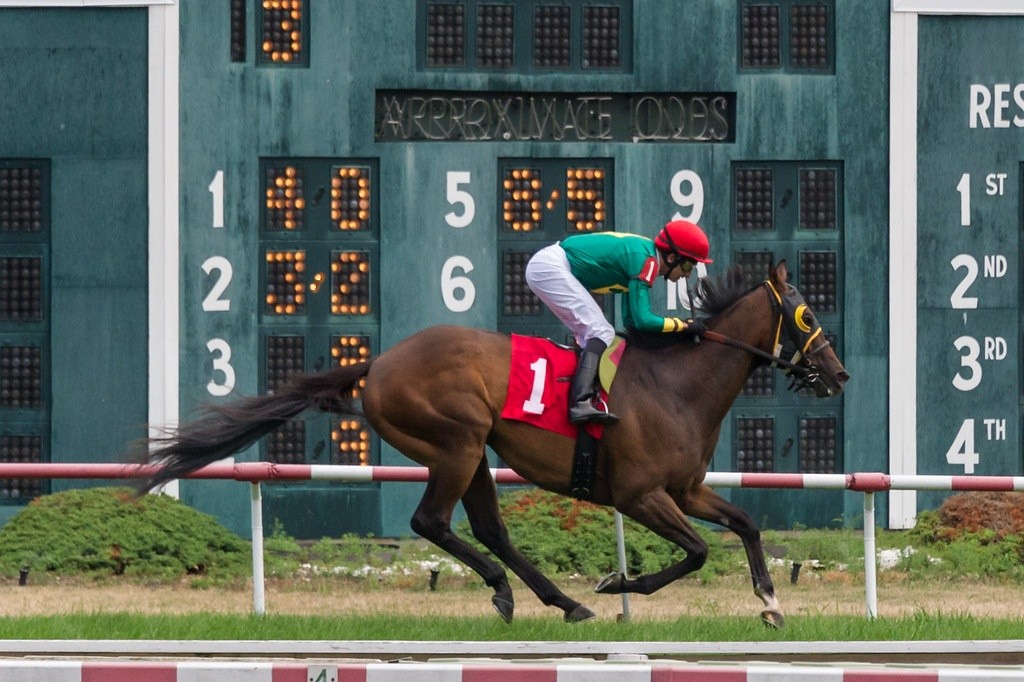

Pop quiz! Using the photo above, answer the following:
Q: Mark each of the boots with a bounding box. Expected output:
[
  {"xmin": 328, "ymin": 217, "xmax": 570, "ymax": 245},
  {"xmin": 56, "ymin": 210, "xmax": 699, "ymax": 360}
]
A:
[{"xmin": 569, "ymin": 351, "xmax": 621, "ymax": 425}]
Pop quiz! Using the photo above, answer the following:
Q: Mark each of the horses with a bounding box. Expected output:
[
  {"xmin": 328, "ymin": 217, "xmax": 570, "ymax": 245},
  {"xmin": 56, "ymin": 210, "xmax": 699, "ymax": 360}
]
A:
[{"xmin": 118, "ymin": 257, "xmax": 851, "ymax": 632}]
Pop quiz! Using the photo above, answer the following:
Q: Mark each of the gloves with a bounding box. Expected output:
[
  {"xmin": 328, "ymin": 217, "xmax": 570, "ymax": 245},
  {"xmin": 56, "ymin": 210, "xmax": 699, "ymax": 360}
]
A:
[{"xmin": 682, "ymin": 318, "xmax": 706, "ymax": 336}]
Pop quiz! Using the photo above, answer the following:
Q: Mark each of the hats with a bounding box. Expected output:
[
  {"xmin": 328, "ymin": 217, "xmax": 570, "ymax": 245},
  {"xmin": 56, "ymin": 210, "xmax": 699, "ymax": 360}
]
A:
[{"xmin": 655, "ymin": 220, "xmax": 713, "ymax": 263}]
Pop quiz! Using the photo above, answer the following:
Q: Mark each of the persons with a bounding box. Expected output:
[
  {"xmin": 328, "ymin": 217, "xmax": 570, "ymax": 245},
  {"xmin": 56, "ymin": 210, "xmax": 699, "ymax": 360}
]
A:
[{"xmin": 525, "ymin": 220, "xmax": 713, "ymax": 426}]
